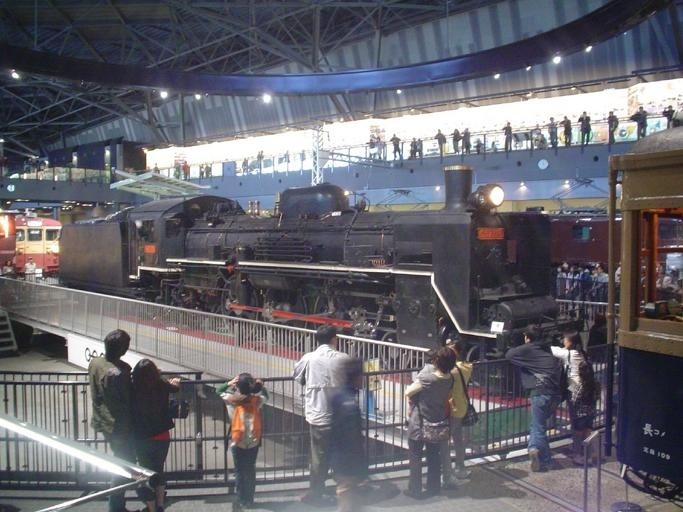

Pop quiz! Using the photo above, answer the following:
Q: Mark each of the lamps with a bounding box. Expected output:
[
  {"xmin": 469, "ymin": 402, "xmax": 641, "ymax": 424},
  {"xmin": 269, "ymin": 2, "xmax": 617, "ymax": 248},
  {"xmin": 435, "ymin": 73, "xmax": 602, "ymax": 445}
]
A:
[{"xmin": 469, "ymin": 183, "xmax": 504, "ymax": 211}]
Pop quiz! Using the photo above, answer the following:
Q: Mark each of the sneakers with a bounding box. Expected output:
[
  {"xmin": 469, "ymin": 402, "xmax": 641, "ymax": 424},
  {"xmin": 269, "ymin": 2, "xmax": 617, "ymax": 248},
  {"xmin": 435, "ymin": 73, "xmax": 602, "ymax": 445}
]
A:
[
  {"xmin": 530, "ymin": 448, "xmax": 542, "ymax": 471},
  {"xmin": 572, "ymin": 455, "xmax": 593, "ymax": 465},
  {"xmin": 403, "ymin": 469, "xmax": 472, "ymax": 500}
]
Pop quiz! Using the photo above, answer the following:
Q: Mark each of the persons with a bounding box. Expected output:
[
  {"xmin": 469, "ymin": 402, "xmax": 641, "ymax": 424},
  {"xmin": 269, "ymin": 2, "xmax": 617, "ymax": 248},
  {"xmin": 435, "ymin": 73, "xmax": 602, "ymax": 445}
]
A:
[
  {"xmin": 549, "ymin": 329, "xmax": 597, "ymax": 456},
  {"xmin": 367, "ymin": 105, "xmax": 676, "ymax": 160},
  {"xmin": 215, "ymin": 372, "xmax": 269, "ymax": 509},
  {"xmin": 153, "ymin": 150, "xmax": 306, "ymax": 181},
  {"xmin": 328, "ymin": 359, "xmax": 369, "ymax": 511},
  {"xmin": 24, "ymin": 257, "xmax": 36, "ymax": 282},
  {"xmin": 131, "ymin": 359, "xmax": 181, "ymax": 512},
  {"xmin": 292, "ymin": 324, "xmax": 350, "ymax": 503},
  {"xmin": 2, "ymin": 259, "xmax": 14, "ymax": 278},
  {"xmin": 505, "ymin": 323, "xmax": 564, "ymax": 473},
  {"xmin": 555, "ymin": 261, "xmax": 682, "ymax": 304},
  {"xmin": 401, "ymin": 316, "xmax": 474, "ymax": 499},
  {"xmin": 87, "ymin": 329, "xmax": 167, "ymax": 512}
]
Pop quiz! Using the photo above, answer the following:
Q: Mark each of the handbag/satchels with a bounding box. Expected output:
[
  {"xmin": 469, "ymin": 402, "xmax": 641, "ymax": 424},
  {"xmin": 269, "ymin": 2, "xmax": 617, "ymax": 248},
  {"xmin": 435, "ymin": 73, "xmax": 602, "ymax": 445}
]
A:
[
  {"xmin": 421, "ymin": 416, "xmax": 451, "ymax": 442},
  {"xmin": 464, "ymin": 404, "xmax": 478, "ymax": 425},
  {"xmin": 170, "ymin": 398, "xmax": 188, "ymax": 418}
]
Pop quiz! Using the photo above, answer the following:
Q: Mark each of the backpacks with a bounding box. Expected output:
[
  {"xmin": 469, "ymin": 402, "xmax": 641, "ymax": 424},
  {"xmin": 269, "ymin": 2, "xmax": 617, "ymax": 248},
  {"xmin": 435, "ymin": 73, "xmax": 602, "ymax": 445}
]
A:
[{"xmin": 231, "ymin": 399, "xmax": 261, "ymax": 449}]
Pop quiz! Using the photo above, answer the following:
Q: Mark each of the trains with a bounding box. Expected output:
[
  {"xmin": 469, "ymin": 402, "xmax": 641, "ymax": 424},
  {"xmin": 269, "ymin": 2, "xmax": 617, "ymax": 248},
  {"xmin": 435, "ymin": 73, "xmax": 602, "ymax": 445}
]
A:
[
  {"xmin": 9, "ymin": 210, "xmax": 64, "ymax": 275},
  {"xmin": 58, "ymin": 162, "xmax": 566, "ymax": 397},
  {"xmin": 603, "ymin": 98, "xmax": 682, "ymax": 504}
]
